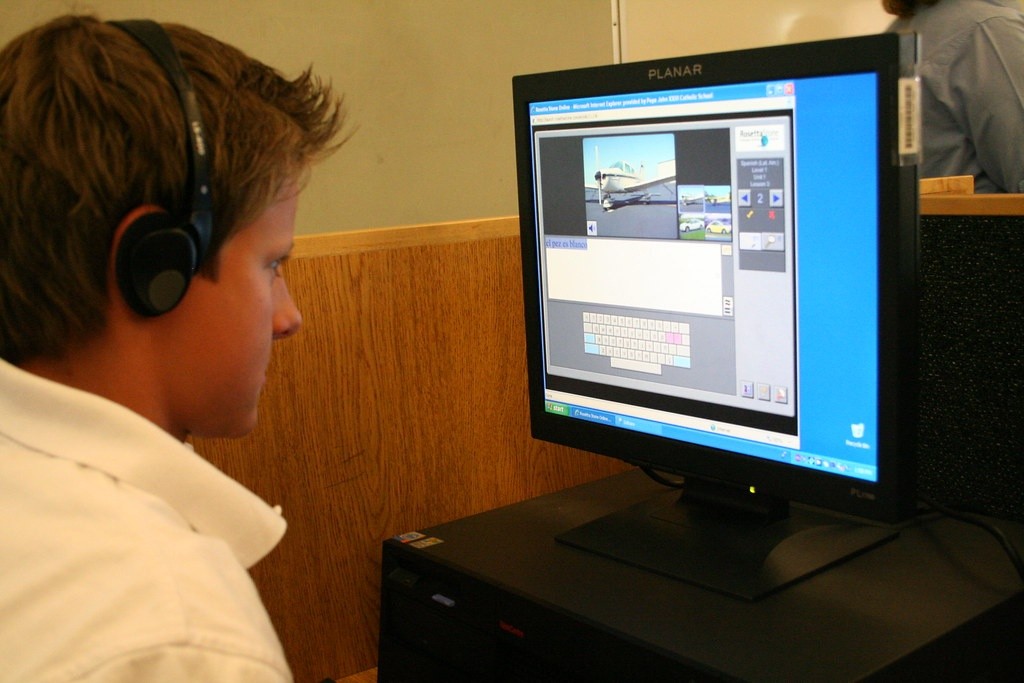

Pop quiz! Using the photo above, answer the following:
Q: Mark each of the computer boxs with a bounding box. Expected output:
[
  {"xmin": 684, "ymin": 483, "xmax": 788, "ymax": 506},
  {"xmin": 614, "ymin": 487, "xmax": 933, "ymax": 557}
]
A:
[{"xmin": 378, "ymin": 472, "xmax": 1024, "ymax": 683}]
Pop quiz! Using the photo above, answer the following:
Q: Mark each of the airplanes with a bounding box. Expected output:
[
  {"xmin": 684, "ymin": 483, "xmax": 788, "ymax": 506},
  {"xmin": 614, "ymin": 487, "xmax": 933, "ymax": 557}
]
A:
[{"xmin": 588, "ymin": 144, "xmax": 676, "ymax": 209}]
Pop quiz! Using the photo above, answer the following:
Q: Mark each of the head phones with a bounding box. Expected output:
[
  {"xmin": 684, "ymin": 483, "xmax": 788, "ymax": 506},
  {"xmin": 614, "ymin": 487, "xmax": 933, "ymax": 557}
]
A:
[{"xmin": 108, "ymin": 18, "xmax": 212, "ymax": 312}]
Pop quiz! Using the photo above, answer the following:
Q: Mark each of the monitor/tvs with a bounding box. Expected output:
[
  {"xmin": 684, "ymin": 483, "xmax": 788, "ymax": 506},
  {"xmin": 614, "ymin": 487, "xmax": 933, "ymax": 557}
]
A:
[{"xmin": 511, "ymin": 29, "xmax": 921, "ymax": 602}]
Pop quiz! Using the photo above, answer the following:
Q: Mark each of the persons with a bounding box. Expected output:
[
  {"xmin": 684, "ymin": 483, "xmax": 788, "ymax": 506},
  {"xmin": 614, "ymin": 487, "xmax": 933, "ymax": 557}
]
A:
[
  {"xmin": 880, "ymin": 0, "xmax": 1024, "ymax": 194},
  {"xmin": 1, "ymin": 15, "xmax": 362, "ymax": 683}
]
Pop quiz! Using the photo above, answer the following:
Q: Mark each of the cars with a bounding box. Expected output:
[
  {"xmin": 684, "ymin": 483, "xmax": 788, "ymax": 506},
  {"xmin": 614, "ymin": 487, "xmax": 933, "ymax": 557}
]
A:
[
  {"xmin": 680, "ymin": 218, "xmax": 706, "ymax": 232},
  {"xmin": 706, "ymin": 220, "xmax": 732, "ymax": 235}
]
